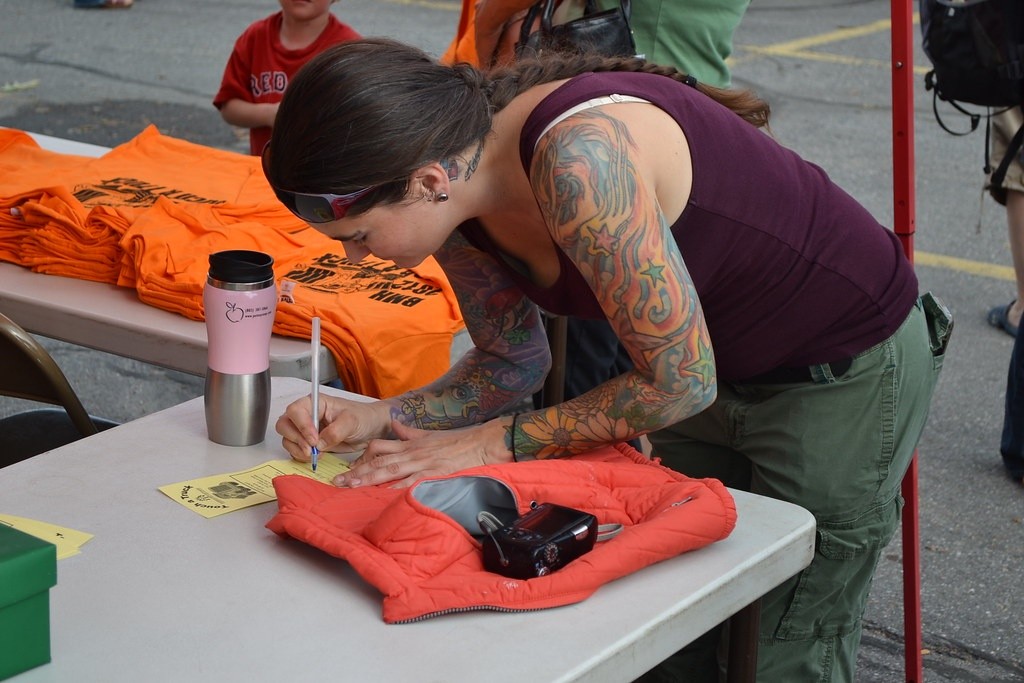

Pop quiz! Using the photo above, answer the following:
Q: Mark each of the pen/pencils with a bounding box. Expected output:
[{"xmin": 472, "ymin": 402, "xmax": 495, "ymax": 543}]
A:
[{"xmin": 312, "ymin": 317, "xmax": 321, "ymax": 471}]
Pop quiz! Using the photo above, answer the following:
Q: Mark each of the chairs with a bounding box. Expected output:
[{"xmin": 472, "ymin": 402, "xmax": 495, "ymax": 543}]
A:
[{"xmin": 0, "ymin": 312, "xmax": 121, "ymax": 469}]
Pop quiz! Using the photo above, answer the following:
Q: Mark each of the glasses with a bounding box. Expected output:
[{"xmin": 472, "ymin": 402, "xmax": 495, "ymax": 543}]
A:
[{"xmin": 261, "ymin": 140, "xmax": 449, "ymax": 224}]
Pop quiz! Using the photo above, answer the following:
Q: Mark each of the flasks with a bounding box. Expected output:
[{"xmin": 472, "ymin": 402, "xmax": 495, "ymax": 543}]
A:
[{"xmin": 202, "ymin": 250, "xmax": 278, "ymax": 446}]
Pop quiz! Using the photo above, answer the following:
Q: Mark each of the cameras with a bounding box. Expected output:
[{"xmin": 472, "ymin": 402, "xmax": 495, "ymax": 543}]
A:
[{"xmin": 483, "ymin": 502, "xmax": 599, "ymax": 580}]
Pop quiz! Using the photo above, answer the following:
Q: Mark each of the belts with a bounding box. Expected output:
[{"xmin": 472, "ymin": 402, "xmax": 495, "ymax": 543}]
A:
[{"xmin": 715, "ymin": 356, "xmax": 853, "ymax": 389}]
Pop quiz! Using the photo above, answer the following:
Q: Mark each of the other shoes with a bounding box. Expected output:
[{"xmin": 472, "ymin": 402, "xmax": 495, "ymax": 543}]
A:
[
  {"xmin": 987, "ymin": 300, "xmax": 1021, "ymax": 336},
  {"xmin": 94, "ymin": 0, "xmax": 135, "ymax": 8}
]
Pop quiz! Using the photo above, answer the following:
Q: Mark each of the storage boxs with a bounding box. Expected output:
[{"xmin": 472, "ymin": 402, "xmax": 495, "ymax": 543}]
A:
[{"xmin": 0, "ymin": 523, "xmax": 57, "ymax": 680}]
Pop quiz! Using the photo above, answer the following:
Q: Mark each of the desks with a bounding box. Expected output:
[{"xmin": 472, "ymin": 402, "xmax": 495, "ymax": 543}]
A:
[
  {"xmin": 0, "ymin": 376, "xmax": 817, "ymax": 683},
  {"xmin": 0, "ymin": 124, "xmax": 339, "ymax": 385}
]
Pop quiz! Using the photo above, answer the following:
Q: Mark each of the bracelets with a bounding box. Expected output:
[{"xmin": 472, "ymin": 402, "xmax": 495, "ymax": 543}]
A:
[{"xmin": 512, "ymin": 411, "xmax": 519, "ymax": 462}]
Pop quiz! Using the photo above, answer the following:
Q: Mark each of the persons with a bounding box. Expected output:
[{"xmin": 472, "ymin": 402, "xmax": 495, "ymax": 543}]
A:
[
  {"xmin": 215, "ymin": 0, "xmax": 365, "ymax": 159},
  {"xmin": 472, "ymin": 0, "xmax": 751, "ymax": 454},
  {"xmin": 986, "ymin": 107, "xmax": 1024, "ymax": 338},
  {"xmin": 260, "ymin": 40, "xmax": 937, "ymax": 682}
]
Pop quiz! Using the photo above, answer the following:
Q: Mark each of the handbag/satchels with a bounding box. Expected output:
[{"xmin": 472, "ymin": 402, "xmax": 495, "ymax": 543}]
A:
[
  {"xmin": 514, "ymin": 0, "xmax": 637, "ymax": 60},
  {"xmin": 919, "ymin": 0, "xmax": 1024, "ymax": 137}
]
